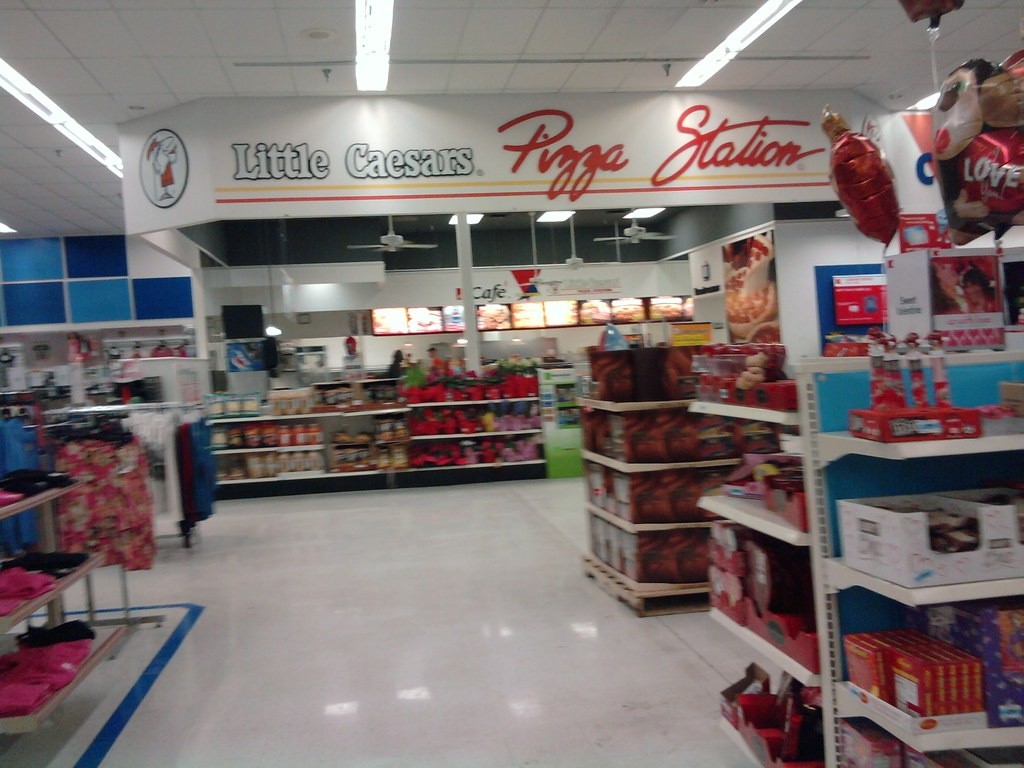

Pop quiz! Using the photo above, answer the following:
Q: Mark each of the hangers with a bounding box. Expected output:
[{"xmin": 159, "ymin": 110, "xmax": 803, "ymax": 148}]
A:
[{"xmin": 1, "ymin": 325, "xmax": 201, "ymax": 443}]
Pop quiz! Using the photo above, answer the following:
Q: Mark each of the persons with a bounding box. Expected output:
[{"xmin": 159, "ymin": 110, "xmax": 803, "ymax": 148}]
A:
[
  {"xmin": 425, "ymin": 346, "xmax": 441, "ymax": 379},
  {"xmin": 385, "ymin": 349, "xmax": 404, "ymax": 385}
]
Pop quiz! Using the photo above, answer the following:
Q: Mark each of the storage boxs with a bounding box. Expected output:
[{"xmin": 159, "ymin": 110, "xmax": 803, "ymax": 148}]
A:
[
  {"xmin": 718, "ymin": 659, "xmax": 825, "ymax": 768},
  {"xmin": 579, "ymin": 344, "xmax": 781, "ymax": 585},
  {"xmin": 721, "ymin": 451, "xmax": 809, "ymax": 531},
  {"xmin": 886, "ymin": 247, "xmax": 1007, "ymax": 353},
  {"xmin": 833, "ymin": 483, "xmax": 1024, "ymax": 766}
]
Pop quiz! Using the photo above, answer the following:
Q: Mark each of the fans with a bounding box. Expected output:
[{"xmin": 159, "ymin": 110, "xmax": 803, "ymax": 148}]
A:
[
  {"xmin": 532, "ymin": 217, "xmax": 622, "ymax": 272},
  {"xmin": 593, "ymin": 208, "xmax": 675, "ymax": 244},
  {"xmin": 347, "ymin": 215, "xmax": 439, "ymax": 253},
  {"xmin": 509, "ymin": 211, "xmax": 563, "ymax": 287}
]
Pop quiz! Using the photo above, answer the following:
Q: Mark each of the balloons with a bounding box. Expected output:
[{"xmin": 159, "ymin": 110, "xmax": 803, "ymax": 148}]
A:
[
  {"xmin": 822, "ymin": 103, "xmax": 902, "ymax": 247},
  {"xmin": 900, "ymin": 0, "xmax": 965, "ymax": 24},
  {"xmin": 931, "ymin": 53, "xmax": 1024, "ymax": 246}
]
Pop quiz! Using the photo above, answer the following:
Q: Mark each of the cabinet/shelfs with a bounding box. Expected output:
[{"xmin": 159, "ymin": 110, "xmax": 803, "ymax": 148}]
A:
[
  {"xmin": 820, "ymin": 413, "xmax": 1023, "ymax": 766},
  {"xmin": 688, "ymin": 349, "xmax": 822, "ymax": 768},
  {"xmin": 576, "ymin": 395, "xmax": 711, "ymax": 619},
  {"xmin": 1, "ymin": 474, "xmax": 128, "ymax": 735},
  {"xmin": 205, "ymin": 396, "xmax": 547, "ymax": 486}
]
